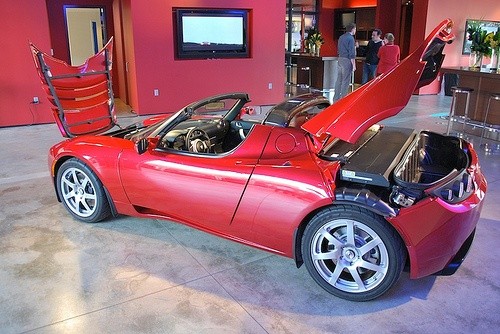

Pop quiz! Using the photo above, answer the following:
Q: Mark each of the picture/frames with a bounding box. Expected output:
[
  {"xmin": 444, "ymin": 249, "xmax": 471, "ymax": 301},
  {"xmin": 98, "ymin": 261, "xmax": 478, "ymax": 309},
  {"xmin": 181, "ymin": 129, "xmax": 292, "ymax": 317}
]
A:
[{"xmin": 462, "ymin": 19, "xmax": 500, "ymax": 55}]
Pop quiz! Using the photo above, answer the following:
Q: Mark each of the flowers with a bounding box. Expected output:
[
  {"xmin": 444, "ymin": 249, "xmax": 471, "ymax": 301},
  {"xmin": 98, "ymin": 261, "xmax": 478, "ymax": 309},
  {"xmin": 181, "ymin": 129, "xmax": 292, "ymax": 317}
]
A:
[
  {"xmin": 464, "ymin": 15, "xmax": 500, "ymax": 67},
  {"xmin": 306, "ymin": 26, "xmax": 325, "ymax": 49}
]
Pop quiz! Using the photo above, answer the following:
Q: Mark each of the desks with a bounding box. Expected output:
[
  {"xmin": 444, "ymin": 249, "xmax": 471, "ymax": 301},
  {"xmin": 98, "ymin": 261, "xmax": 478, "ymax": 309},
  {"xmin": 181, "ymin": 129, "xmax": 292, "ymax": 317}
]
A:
[
  {"xmin": 439, "ymin": 66, "xmax": 500, "ymax": 142},
  {"xmin": 287, "ymin": 54, "xmax": 354, "ymax": 99}
]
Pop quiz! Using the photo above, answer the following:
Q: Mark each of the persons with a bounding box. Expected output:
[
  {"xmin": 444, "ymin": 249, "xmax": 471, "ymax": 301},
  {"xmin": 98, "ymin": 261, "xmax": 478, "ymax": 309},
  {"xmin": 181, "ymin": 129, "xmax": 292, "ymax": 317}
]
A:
[
  {"xmin": 333, "ymin": 22, "xmax": 356, "ymax": 103},
  {"xmin": 374, "ymin": 33, "xmax": 400, "ymax": 77},
  {"xmin": 356, "ymin": 29, "xmax": 383, "ymax": 85}
]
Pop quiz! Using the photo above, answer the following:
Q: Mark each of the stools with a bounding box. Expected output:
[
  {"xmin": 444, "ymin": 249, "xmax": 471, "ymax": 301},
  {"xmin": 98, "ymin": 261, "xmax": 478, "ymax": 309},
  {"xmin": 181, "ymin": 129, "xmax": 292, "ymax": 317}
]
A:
[
  {"xmin": 479, "ymin": 93, "xmax": 500, "ymax": 153},
  {"xmin": 285, "ymin": 63, "xmax": 293, "ymax": 85},
  {"xmin": 446, "ymin": 87, "xmax": 474, "ymax": 139},
  {"xmin": 301, "ymin": 66, "xmax": 316, "ymax": 92}
]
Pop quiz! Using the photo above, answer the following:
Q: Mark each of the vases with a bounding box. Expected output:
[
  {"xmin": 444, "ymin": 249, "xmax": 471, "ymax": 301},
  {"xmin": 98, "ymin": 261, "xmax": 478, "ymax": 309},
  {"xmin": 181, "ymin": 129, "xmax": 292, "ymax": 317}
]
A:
[
  {"xmin": 468, "ymin": 51, "xmax": 482, "ymax": 72},
  {"xmin": 492, "ymin": 51, "xmax": 500, "ymax": 74},
  {"xmin": 309, "ymin": 43, "xmax": 319, "ymax": 57}
]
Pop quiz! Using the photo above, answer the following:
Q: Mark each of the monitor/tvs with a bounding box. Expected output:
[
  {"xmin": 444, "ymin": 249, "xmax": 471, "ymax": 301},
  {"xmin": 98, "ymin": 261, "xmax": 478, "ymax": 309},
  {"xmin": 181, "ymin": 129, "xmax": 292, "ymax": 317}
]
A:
[{"xmin": 176, "ymin": 9, "xmax": 248, "ymax": 58}]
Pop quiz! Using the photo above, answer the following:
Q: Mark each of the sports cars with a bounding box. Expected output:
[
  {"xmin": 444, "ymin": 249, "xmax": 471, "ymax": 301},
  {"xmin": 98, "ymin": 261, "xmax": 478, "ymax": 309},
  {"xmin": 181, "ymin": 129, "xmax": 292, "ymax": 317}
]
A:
[{"xmin": 29, "ymin": 17, "xmax": 489, "ymax": 301}]
